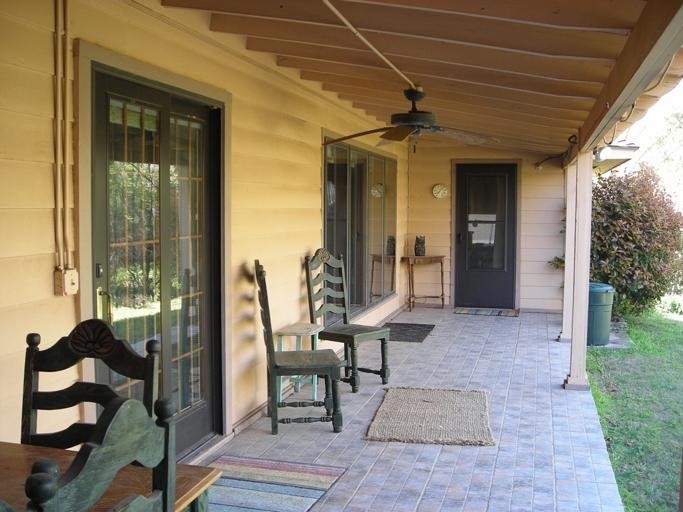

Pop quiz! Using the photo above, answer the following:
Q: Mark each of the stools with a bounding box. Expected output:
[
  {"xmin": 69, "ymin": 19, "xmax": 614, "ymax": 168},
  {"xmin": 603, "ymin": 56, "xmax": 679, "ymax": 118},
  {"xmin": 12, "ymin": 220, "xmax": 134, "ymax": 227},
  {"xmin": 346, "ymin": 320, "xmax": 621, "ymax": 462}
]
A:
[{"xmin": 275, "ymin": 322, "xmax": 327, "ymax": 404}]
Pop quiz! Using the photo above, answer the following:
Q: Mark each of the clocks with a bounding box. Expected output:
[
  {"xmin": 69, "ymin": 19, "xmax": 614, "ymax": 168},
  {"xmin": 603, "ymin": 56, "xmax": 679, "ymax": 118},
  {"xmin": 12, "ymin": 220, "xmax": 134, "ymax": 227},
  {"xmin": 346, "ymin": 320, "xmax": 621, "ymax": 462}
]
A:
[
  {"xmin": 431, "ymin": 184, "xmax": 449, "ymax": 200},
  {"xmin": 370, "ymin": 181, "xmax": 387, "ymax": 199}
]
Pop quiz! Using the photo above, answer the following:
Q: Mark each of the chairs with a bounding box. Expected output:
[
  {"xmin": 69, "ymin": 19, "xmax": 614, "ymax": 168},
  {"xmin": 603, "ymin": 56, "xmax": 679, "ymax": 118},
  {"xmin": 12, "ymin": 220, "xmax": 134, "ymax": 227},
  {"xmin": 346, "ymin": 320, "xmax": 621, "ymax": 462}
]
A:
[
  {"xmin": 14, "ymin": 316, "xmax": 166, "ymax": 455},
  {"xmin": 300, "ymin": 243, "xmax": 391, "ymax": 394},
  {"xmin": 22, "ymin": 394, "xmax": 178, "ymax": 512},
  {"xmin": 251, "ymin": 257, "xmax": 345, "ymax": 437}
]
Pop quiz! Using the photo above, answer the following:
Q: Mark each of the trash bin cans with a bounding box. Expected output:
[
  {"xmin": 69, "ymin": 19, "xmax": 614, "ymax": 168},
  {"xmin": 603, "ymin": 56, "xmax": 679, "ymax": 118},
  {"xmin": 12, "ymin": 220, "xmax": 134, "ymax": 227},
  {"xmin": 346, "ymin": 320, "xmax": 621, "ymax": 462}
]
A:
[{"xmin": 587, "ymin": 282, "xmax": 616, "ymax": 346}]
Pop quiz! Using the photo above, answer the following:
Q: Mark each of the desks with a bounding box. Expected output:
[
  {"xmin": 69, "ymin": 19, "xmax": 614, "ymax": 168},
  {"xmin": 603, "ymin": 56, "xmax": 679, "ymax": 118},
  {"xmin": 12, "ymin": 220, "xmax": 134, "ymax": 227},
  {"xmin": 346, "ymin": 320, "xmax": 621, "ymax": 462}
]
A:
[
  {"xmin": 402, "ymin": 254, "xmax": 448, "ymax": 312},
  {"xmin": 0, "ymin": 432, "xmax": 223, "ymax": 512},
  {"xmin": 365, "ymin": 254, "xmax": 397, "ymax": 302}
]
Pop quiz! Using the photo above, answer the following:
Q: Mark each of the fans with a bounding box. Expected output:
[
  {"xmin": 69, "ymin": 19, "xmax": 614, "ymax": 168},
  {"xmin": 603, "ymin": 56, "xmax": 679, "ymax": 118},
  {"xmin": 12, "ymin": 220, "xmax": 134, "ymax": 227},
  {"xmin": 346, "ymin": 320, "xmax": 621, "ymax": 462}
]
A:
[{"xmin": 317, "ymin": 86, "xmax": 500, "ymax": 156}]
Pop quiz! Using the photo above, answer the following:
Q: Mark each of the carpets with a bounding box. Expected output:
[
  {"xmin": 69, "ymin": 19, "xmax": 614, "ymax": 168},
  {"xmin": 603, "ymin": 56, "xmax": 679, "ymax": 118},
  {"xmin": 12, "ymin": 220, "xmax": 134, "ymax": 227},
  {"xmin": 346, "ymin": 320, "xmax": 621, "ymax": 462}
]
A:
[
  {"xmin": 362, "ymin": 387, "xmax": 497, "ymax": 447},
  {"xmin": 451, "ymin": 305, "xmax": 521, "ymax": 318},
  {"xmin": 378, "ymin": 320, "xmax": 436, "ymax": 345},
  {"xmin": 186, "ymin": 450, "xmax": 347, "ymax": 512}
]
